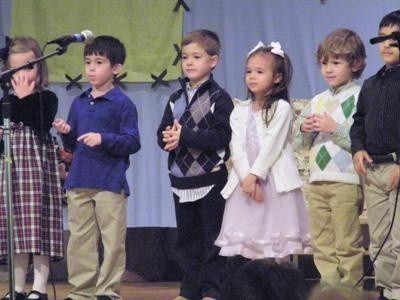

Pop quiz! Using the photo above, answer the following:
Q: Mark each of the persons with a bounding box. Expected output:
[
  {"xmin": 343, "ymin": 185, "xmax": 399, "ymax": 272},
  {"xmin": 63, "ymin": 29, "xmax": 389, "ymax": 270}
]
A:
[
  {"xmin": 0, "ymin": 36, "xmax": 65, "ymax": 300},
  {"xmin": 214, "ymin": 42, "xmax": 314, "ymax": 268},
  {"xmin": 156, "ymin": 29, "xmax": 235, "ymax": 300},
  {"xmin": 51, "ymin": 36, "xmax": 140, "ymax": 300},
  {"xmin": 294, "ymin": 29, "xmax": 366, "ymax": 298},
  {"xmin": 201, "ymin": 257, "xmax": 372, "ymax": 299},
  {"xmin": 349, "ymin": 11, "xmax": 400, "ymax": 300}
]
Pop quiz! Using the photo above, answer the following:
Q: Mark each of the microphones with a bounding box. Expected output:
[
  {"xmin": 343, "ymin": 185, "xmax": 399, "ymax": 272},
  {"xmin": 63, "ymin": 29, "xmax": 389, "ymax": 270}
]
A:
[{"xmin": 47, "ymin": 30, "xmax": 94, "ymax": 45}]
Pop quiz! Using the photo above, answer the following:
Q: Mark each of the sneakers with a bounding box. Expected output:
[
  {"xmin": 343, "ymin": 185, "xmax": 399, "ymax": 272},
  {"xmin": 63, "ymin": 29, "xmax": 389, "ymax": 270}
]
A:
[{"xmin": 0, "ymin": 290, "xmax": 49, "ymax": 300}]
